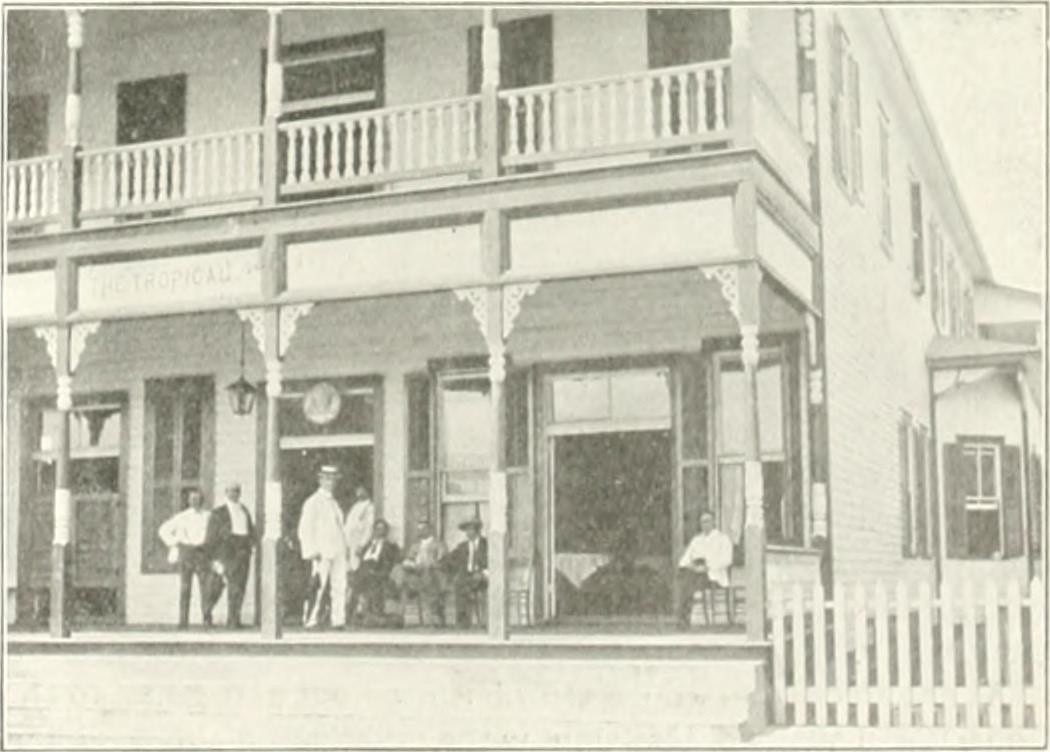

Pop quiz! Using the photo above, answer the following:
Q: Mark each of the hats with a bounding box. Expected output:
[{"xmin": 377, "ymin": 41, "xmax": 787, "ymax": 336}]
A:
[
  {"xmin": 317, "ymin": 463, "xmax": 343, "ymax": 480},
  {"xmin": 457, "ymin": 517, "xmax": 483, "ymax": 530}
]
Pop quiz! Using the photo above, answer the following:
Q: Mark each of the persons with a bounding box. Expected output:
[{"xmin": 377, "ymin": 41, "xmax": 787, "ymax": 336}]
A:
[
  {"xmin": 159, "ymin": 492, "xmax": 215, "ymax": 629},
  {"xmin": 298, "ymin": 466, "xmax": 348, "ymax": 631},
  {"xmin": 344, "ymin": 487, "xmax": 375, "ymax": 614},
  {"xmin": 674, "ymin": 512, "xmax": 732, "ymax": 632},
  {"xmin": 347, "ymin": 519, "xmax": 487, "ymax": 629},
  {"xmin": 203, "ymin": 485, "xmax": 253, "ymax": 624}
]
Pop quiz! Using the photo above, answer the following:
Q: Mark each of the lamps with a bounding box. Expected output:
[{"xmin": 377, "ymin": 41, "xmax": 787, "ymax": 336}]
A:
[{"xmin": 224, "ymin": 324, "xmax": 257, "ymax": 415}]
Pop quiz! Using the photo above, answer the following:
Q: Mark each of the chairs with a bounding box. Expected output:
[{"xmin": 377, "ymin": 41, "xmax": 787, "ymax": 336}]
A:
[
  {"xmin": 503, "ymin": 550, "xmax": 535, "ymax": 629},
  {"xmin": 690, "ymin": 544, "xmax": 747, "ymax": 626}
]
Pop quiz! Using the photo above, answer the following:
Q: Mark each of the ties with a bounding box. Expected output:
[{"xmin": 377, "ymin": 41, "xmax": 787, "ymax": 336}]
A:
[{"xmin": 371, "ymin": 539, "xmax": 379, "ymax": 554}]
